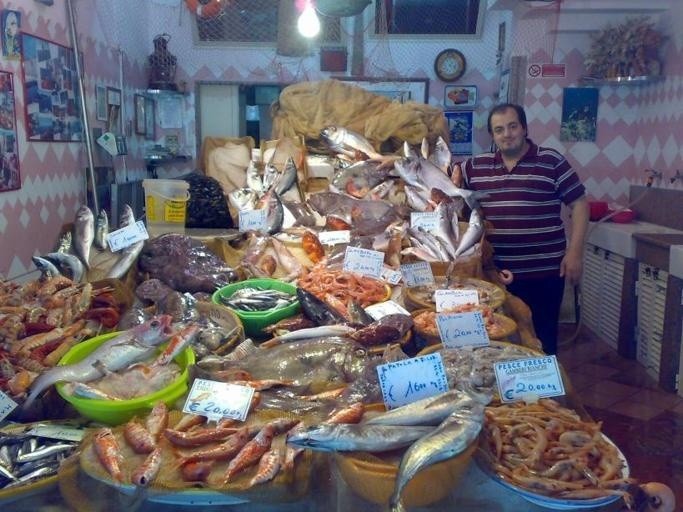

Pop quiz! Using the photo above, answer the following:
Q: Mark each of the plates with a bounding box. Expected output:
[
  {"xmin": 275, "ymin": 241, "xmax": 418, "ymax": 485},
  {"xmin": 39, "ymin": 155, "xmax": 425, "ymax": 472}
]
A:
[
  {"xmin": 479, "ymin": 430, "xmax": 630, "ymax": 511},
  {"xmin": 79, "ymin": 426, "xmax": 296, "ymax": 507}
]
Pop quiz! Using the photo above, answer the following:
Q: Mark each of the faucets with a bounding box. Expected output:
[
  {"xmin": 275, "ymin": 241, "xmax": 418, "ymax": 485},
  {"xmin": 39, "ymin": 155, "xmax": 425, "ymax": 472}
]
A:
[
  {"xmin": 668, "ymin": 169, "xmax": 683, "ymax": 183},
  {"xmin": 644, "ymin": 169, "xmax": 662, "ymax": 179}
]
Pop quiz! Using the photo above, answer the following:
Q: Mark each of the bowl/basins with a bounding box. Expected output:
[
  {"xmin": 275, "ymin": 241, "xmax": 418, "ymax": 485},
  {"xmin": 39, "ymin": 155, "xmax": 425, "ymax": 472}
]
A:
[
  {"xmin": 56, "ymin": 279, "xmax": 302, "ymax": 423},
  {"xmin": 609, "ymin": 209, "xmax": 637, "ymax": 223}
]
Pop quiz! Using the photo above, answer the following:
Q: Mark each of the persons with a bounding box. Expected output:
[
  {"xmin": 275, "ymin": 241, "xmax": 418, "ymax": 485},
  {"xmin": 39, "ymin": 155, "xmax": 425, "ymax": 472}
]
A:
[{"xmin": 459, "ymin": 103, "xmax": 591, "ymax": 356}]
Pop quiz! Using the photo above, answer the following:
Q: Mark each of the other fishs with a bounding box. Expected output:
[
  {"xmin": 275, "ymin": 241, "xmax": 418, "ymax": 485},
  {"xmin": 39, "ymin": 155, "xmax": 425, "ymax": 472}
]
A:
[{"xmin": 0, "ymin": 124, "xmax": 496, "ymax": 512}]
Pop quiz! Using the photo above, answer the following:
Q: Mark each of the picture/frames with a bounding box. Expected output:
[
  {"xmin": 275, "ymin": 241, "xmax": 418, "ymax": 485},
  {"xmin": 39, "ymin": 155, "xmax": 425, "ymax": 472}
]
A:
[
  {"xmin": 330, "ymin": 75, "xmax": 429, "ymax": 105},
  {"xmin": 96, "ymin": 83, "xmax": 155, "ymax": 139},
  {"xmin": 1, "ymin": 31, "xmax": 88, "ymax": 192}
]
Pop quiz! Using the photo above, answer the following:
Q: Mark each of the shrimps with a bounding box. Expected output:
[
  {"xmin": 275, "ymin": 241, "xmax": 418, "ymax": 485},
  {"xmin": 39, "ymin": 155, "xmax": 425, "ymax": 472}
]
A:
[{"xmin": 477, "ymin": 397, "xmax": 640, "ymax": 512}]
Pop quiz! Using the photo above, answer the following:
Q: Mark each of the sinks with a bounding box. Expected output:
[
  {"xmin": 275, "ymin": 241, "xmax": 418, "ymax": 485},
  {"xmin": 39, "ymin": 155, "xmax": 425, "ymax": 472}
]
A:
[{"xmin": 603, "ymin": 221, "xmax": 682, "ymax": 258}]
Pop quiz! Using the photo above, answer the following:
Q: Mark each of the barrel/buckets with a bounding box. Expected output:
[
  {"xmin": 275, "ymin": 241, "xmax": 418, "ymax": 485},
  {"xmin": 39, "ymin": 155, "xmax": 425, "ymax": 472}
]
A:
[{"xmin": 142, "ymin": 179, "xmax": 191, "ymax": 236}]
